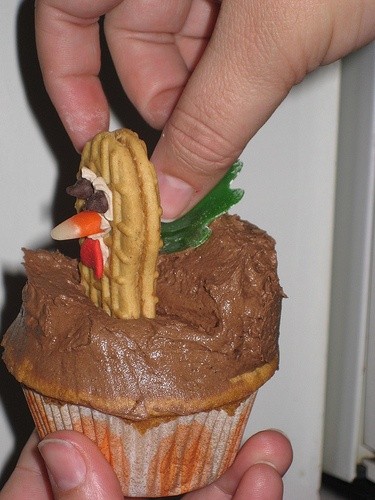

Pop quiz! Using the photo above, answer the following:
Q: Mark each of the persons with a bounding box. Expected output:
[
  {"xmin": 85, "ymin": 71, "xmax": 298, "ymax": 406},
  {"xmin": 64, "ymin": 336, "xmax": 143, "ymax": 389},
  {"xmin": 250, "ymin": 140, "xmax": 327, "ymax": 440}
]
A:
[{"xmin": 1, "ymin": 0, "xmax": 375, "ymax": 500}]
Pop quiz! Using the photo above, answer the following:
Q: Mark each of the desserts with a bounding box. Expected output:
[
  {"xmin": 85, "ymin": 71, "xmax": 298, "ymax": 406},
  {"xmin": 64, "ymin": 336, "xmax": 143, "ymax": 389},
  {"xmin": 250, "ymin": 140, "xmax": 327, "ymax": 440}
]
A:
[{"xmin": 1, "ymin": 211, "xmax": 288, "ymax": 500}]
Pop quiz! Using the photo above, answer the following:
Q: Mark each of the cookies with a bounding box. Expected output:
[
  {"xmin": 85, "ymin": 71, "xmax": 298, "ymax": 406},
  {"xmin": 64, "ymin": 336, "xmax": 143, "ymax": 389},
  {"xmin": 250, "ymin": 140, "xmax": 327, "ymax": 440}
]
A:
[{"xmin": 67, "ymin": 125, "xmax": 164, "ymax": 322}]
